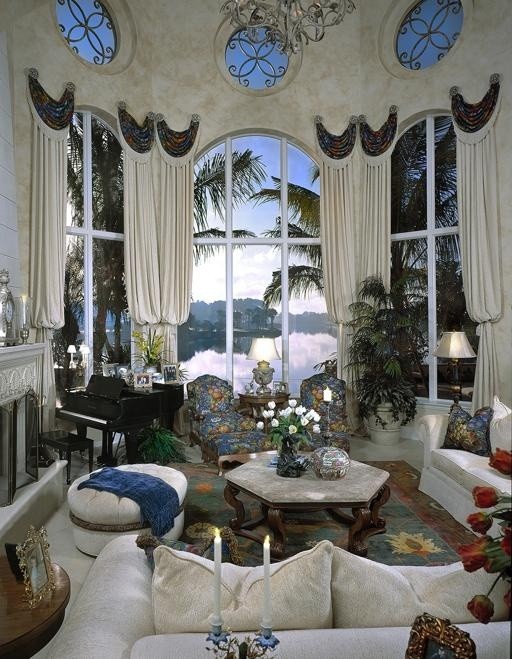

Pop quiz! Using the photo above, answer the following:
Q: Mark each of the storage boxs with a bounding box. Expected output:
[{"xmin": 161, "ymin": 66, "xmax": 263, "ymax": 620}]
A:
[{"xmin": 309, "ymin": 445, "xmax": 352, "ymax": 482}]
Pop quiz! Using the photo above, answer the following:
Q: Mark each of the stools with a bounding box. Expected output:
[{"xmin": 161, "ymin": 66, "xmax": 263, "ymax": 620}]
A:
[{"xmin": 37, "ymin": 428, "xmax": 95, "ymax": 485}]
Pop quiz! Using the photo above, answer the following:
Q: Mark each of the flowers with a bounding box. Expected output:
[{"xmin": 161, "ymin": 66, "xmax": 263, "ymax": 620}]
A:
[
  {"xmin": 122, "ymin": 319, "xmax": 174, "ymax": 370},
  {"xmin": 460, "ymin": 446, "xmax": 510, "ymax": 626},
  {"xmin": 256, "ymin": 397, "xmax": 322, "ymax": 447}
]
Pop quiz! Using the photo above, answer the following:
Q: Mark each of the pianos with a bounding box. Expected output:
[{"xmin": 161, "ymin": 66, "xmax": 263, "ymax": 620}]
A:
[{"xmin": 56, "ymin": 375, "xmax": 185, "ymax": 468}]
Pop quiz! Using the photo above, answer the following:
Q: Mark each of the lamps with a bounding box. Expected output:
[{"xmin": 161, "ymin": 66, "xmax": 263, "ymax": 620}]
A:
[
  {"xmin": 245, "ymin": 336, "xmax": 282, "ymax": 395},
  {"xmin": 218, "ymin": 0, "xmax": 354, "ymax": 60},
  {"xmin": 66, "ymin": 343, "xmax": 92, "ymax": 369},
  {"xmin": 431, "ymin": 329, "xmax": 477, "ymax": 404}
]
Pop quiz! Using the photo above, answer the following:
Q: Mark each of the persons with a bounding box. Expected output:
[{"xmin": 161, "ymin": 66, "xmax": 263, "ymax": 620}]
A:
[
  {"xmin": 30, "ymin": 555, "xmax": 41, "ymax": 595},
  {"xmin": 138, "ymin": 376, "xmax": 147, "ymax": 385}
]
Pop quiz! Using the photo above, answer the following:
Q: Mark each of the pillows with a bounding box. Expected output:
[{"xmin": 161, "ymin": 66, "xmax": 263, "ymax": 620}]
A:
[
  {"xmin": 149, "ymin": 538, "xmax": 336, "ymax": 636},
  {"xmin": 326, "ymin": 542, "xmax": 510, "ymax": 626},
  {"xmin": 439, "ymin": 401, "xmax": 493, "ymax": 456},
  {"xmin": 485, "ymin": 392, "xmax": 512, "ymax": 453}
]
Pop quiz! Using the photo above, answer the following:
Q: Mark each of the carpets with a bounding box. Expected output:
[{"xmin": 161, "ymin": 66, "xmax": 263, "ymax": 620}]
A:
[{"xmin": 167, "ymin": 460, "xmax": 479, "ymax": 569}]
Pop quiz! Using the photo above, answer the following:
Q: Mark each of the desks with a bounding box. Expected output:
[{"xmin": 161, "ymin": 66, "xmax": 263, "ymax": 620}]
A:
[
  {"xmin": 236, "ymin": 390, "xmax": 292, "ymax": 433},
  {"xmin": 1, "ymin": 553, "xmax": 73, "ymax": 657}
]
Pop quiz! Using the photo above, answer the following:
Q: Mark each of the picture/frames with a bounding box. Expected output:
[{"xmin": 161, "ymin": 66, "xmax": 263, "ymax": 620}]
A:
[
  {"xmin": 133, "ymin": 371, "xmax": 154, "ymax": 388},
  {"xmin": 12, "ymin": 523, "xmax": 57, "ymax": 608},
  {"xmin": 402, "ymin": 611, "xmax": 477, "ymax": 659},
  {"xmin": 160, "ymin": 363, "xmax": 179, "ymax": 384},
  {"xmin": 102, "ymin": 362, "xmax": 120, "ymax": 378},
  {"xmin": 5, "ymin": 541, "xmax": 28, "ymax": 585},
  {"xmin": 115, "ymin": 364, "xmax": 130, "ymax": 381}
]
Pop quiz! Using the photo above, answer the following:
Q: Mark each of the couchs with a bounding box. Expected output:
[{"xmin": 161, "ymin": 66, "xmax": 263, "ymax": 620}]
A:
[
  {"xmin": 66, "ymin": 461, "xmax": 188, "ymax": 560},
  {"xmin": 412, "ymin": 407, "xmax": 510, "ymax": 541},
  {"xmin": 48, "ymin": 529, "xmax": 511, "ymax": 658}
]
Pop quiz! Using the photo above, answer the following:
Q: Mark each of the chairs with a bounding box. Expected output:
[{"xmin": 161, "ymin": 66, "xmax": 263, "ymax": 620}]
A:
[
  {"xmin": 297, "ymin": 373, "xmax": 351, "ymax": 456},
  {"xmin": 186, "ymin": 371, "xmax": 277, "ymax": 478}
]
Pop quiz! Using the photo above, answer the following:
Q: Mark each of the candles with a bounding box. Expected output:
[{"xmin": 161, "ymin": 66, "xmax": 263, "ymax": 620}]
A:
[
  {"xmin": 258, "ymin": 531, "xmax": 275, "ymax": 627},
  {"xmin": 20, "ymin": 294, "xmax": 29, "ymax": 325},
  {"xmin": 208, "ymin": 526, "xmax": 226, "ymax": 621},
  {"xmin": 323, "ymin": 385, "xmax": 333, "ymax": 402}
]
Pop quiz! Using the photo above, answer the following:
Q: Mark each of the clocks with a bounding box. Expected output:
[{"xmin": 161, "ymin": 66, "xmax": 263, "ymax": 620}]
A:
[{"xmin": 0, "ymin": 266, "xmax": 20, "ymax": 348}]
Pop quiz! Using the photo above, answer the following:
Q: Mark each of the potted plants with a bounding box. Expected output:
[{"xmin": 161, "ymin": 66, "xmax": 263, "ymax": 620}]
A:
[{"xmin": 339, "ymin": 272, "xmax": 441, "ymax": 445}]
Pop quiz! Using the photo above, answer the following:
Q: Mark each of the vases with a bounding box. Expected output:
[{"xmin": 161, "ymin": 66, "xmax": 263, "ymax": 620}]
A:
[
  {"xmin": 143, "ymin": 366, "xmax": 158, "ymax": 376},
  {"xmin": 274, "ymin": 433, "xmax": 305, "ymax": 478}
]
